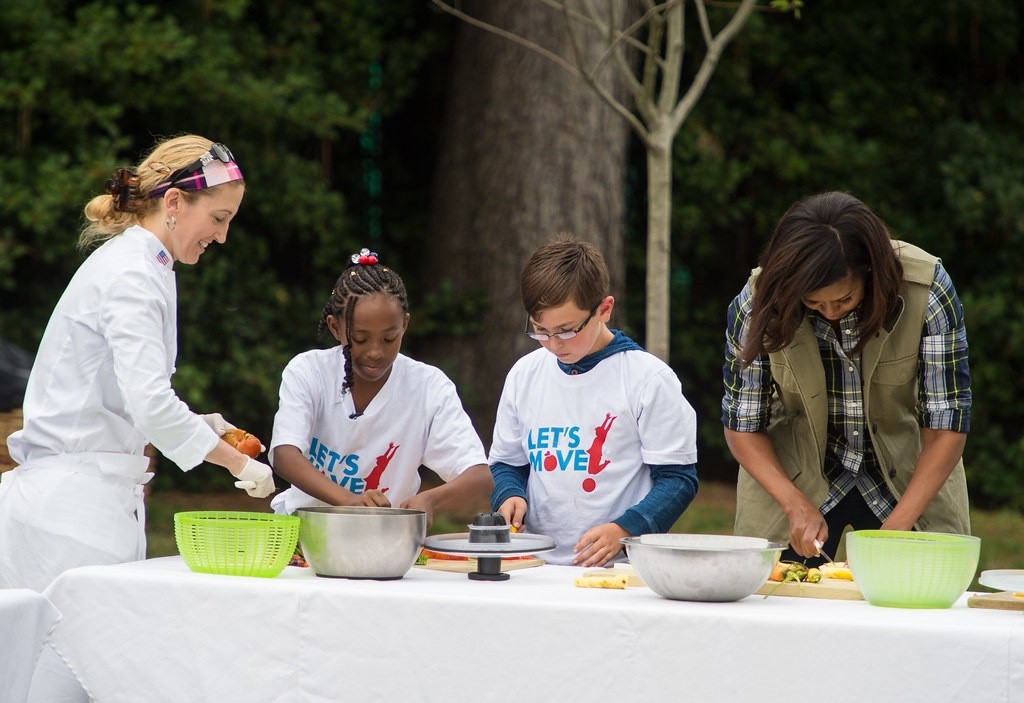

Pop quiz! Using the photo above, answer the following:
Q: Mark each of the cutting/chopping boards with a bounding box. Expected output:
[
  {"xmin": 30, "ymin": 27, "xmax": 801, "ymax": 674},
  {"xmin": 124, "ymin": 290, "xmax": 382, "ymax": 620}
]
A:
[
  {"xmin": 967, "ymin": 591, "xmax": 1024, "ymax": 611},
  {"xmin": 421, "ymin": 558, "xmax": 545, "ymax": 573},
  {"xmin": 581, "ymin": 563, "xmax": 864, "ymax": 600}
]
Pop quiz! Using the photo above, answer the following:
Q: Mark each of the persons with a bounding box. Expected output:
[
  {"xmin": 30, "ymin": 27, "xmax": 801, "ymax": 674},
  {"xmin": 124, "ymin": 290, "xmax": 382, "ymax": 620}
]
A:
[
  {"xmin": 489, "ymin": 232, "xmax": 699, "ymax": 568},
  {"xmin": 720, "ymin": 190, "xmax": 973, "ymax": 569},
  {"xmin": 0, "ymin": 134, "xmax": 276, "ymax": 701},
  {"xmin": 267, "ymin": 247, "xmax": 495, "ymax": 533}
]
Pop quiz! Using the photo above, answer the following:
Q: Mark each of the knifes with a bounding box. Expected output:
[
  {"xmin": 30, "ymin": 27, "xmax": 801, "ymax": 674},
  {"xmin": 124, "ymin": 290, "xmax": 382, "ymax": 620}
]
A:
[{"xmin": 814, "ymin": 539, "xmax": 833, "ymax": 564}]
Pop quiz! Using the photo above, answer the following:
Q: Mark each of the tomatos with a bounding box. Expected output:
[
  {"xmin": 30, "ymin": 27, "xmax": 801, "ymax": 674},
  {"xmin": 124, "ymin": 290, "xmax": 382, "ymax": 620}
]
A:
[{"xmin": 221, "ymin": 428, "xmax": 261, "ymax": 458}]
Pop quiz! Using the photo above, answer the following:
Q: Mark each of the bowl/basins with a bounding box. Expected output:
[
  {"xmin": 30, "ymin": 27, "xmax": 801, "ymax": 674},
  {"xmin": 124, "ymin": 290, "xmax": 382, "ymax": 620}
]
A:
[
  {"xmin": 620, "ymin": 536, "xmax": 788, "ymax": 601},
  {"xmin": 297, "ymin": 506, "xmax": 427, "ymax": 580}
]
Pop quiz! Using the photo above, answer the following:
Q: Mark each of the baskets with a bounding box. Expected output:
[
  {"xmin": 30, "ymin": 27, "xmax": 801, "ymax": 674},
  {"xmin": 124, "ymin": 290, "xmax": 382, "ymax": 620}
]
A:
[
  {"xmin": 174, "ymin": 510, "xmax": 300, "ymax": 578},
  {"xmin": 844, "ymin": 529, "xmax": 980, "ymax": 610}
]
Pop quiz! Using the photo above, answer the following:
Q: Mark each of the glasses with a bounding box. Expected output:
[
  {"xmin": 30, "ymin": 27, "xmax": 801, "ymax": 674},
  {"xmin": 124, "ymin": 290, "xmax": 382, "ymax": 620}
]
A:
[
  {"xmin": 163, "ymin": 142, "xmax": 234, "ymax": 198},
  {"xmin": 524, "ymin": 296, "xmax": 606, "ymax": 341}
]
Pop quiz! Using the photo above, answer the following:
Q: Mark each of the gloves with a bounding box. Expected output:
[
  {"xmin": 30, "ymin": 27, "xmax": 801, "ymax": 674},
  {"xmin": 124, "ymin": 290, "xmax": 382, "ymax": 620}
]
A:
[
  {"xmin": 228, "ymin": 453, "xmax": 276, "ymax": 499},
  {"xmin": 199, "ymin": 412, "xmax": 266, "ymax": 452}
]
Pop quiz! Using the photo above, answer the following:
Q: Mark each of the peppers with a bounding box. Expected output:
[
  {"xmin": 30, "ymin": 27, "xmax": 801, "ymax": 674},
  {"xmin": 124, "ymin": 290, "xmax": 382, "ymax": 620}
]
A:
[{"xmin": 769, "ymin": 561, "xmax": 854, "ymax": 582}]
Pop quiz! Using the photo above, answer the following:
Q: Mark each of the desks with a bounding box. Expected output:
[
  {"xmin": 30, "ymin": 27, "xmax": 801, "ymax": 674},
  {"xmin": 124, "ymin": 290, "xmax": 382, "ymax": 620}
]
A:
[{"xmin": 40, "ymin": 556, "xmax": 1024, "ymax": 703}]
